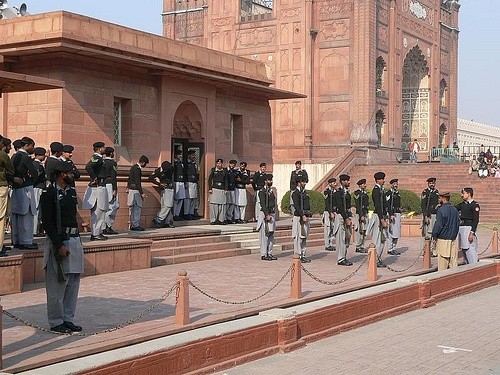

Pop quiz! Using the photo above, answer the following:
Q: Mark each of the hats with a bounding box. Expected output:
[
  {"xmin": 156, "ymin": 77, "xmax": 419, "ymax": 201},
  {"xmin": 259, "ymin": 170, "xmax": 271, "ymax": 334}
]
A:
[
  {"xmin": 461, "ymin": 188, "xmax": 473, "ymax": 192},
  {"xmin": 328, "ymin": 178, "xmax": 336, "ymax": 183},
  {"xmin": 263, "ymin": 174, "xmax": 273, "ymax": 180},
  {"xmin": 215, "ymin": 159, "xmax": 266, "ymax": 167},
  {"xmin": 439, "ymin": 191, "xmax": 450, "ymax": 196},
  {"xmin": 0, "ymin": 134, "xmax": 172, "ymax": 173},
  {"xmin": 374, "ymin": 172, "xmax": 385, "ymax": 179},
  {"xmin": 426, "ymin": 178, "xmax": 436, "ymax": 182},
  {"xmin": 339, "ymin": 175, "xmax": 350, "ymax": 180},
  {"xmin": 295, "ymin": 161, "xmax": 301, "ymax": 164},
  {"xmin": 389, "ymin": 179, "xmax": 398, "ymax": 184},
  {"xmin": 296, "ymin": 175, "xmax": 307, "ymax": 181},
  {"xmin": 357, "ymin": 179, "xmax": 366, "ymax": 185},
  {"xmin": 176, "ymin": 150, "xmax": 196, "ymax": 155}
]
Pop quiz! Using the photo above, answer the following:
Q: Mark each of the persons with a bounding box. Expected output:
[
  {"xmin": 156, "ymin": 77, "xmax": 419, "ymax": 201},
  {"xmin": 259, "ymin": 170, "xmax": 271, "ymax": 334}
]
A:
[
  {"xmin": 256, "ymin": 174, "xmax": 277, "ymax": 261},
  {"xmin": 409, "ymin": 139, "xmax": 421, "ymax": 163},
  {"xmin": 39, "ymin": 164, "xmax": 84, "ymax": 334},
  {"xmin": 126, "ymin": 155, "xmax": 149, "ymax": 231},
  {"xmin": 148, "ymin": 160, "xmax": 175, "ymax": 229},
  {"xmin": 421, "ymin": 177, "xmax": 481, "ymax": 271},
  {"xmin": 452, "ymin": 141, "xmax": 459, "ymax": 156},
  {"xmin": 173, "ymin": 149, "xmax": 197, "ymax": 221},
  {"xmin": 82, "ymin": 142, "xmax": 120, "ymax": 240},
  {"xmin": 0, "ymin": 134, "xmax": 81, "ymax": 257},
  {"xmin": 289, "ymin": 161, "xmax": 313, "ymax": 263},
  {"xmin": 322, "ymin": 171, "xmax": 402, "ymax": 267},
  {"xmin": 208, "ymin": 159, "xmax": 267, "ymax": 225},
  {"xmin": 468, "ymin": 149, "xmax": 500, "ymax": 177}
]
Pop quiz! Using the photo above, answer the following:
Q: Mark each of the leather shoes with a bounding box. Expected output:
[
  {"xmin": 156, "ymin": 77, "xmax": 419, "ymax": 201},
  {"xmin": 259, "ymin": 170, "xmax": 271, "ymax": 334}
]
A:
[
  {"xmin": 64, "ymin": 321, "xmax": 82, "ymax": 332},
  {"xmin": 173, "ymin": 213, "xmax": 200, "ymax": 221},
  {"xmin": 51, "ymin": 323, "xmax": 71, "ymax": 333},
  {"xmin": 325, "ymin": 246, "xmax": 401, "ymax": 268},
  {"xmin": 262, "ymin": 256, "xmax": 272, "ymax": 260},
  {"xmin": 300, "ymin": 258, "xmax": 305, "ymax": 263},
  {"xmin": 303, "ymin": 257, "xmax": 310, "ymax": 262},
  {"xmin": 269, "ymin": 254, "xmax": 277, "ymax": 260},
  {"xmin": 0, "ymin": 233, "xmax": 46, "ymax": 257},
  {"xmin": 90, "ymin": 219, "xmax": 175, "ymax": 241},
  {"xmin": 211, "ymin": 218, "xmax": 245, "ymax": 225}
]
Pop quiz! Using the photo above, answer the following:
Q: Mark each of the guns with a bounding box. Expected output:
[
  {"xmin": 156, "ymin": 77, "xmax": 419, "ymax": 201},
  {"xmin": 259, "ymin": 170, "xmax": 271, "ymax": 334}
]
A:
[
  {"xmin": 379, "ymin": 186, "xmax": 389, "ymax": 242},
  {"xmin": 299, "ymin": 186, "xmax": 308, "ymax": 236},
  {"xmin": 388, "ymin": 185, "xmax": 395, "ymax": 232},
  {"xmin": 264, "ymin": 178, "xmax": 273, "ymax": 236},
  {"xmin": 358, "ymin": 188, "xmax": 365, "ymax": 234},
  {"xmin": 53, "ymin": 171, "xmax": 70, "ymax": 283},
  {"xmin": 329, "ymin": 186, "xmax": 335, "ymax": 231},
  {"xmin": 421, "ymin": 188, "xmax": 431, "ymax": 236}
]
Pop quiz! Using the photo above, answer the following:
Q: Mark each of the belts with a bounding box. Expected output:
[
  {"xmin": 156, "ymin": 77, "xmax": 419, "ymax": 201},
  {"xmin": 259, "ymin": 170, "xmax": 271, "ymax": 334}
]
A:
[{"xmin": 66, "ymin": 227, "xmax": 78, "ymax": 234}]
